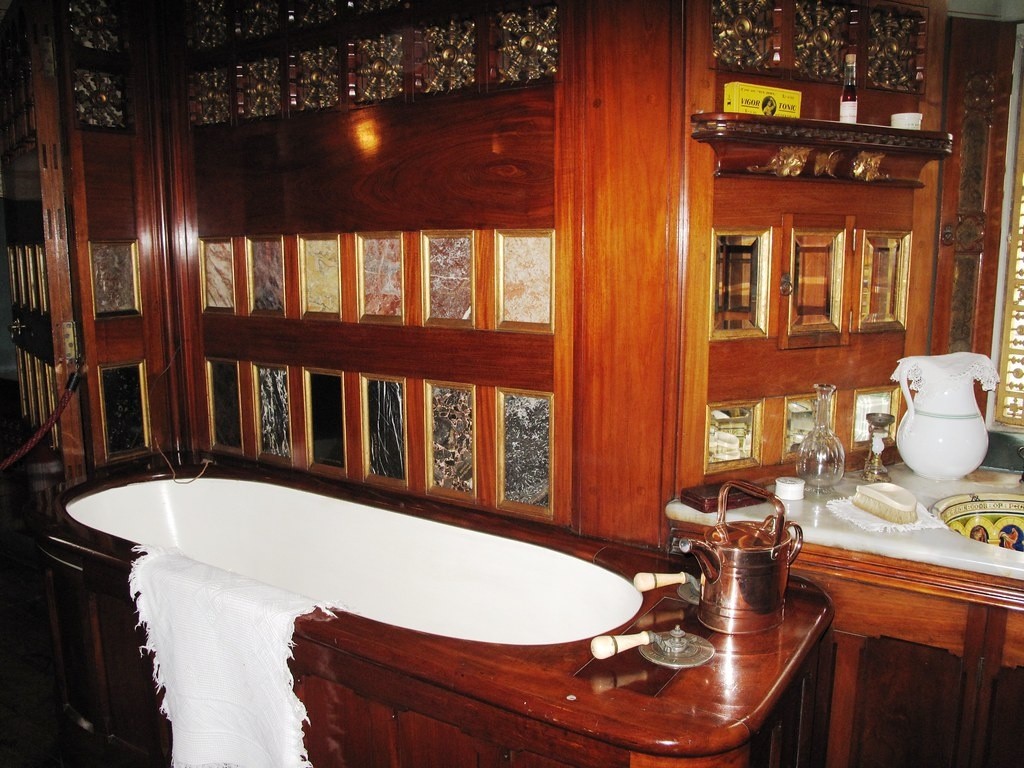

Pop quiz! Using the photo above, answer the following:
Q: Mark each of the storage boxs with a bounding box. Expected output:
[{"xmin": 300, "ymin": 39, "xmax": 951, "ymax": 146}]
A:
[{"xmin": 724, "ymin": 81, "xmax": 802, "ymax": 120}]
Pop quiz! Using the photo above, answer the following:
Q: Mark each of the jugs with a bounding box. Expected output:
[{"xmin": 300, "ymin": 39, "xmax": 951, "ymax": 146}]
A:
[{"xmin": 680, "ymin": 480, "xmax": 802, "ymax": 634}]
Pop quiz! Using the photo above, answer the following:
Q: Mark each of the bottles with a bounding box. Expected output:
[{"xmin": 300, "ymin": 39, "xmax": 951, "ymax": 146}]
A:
[
  {"xmin": 840, "ymin": 54, "xmax": 858, "ymax": 123},
  {"xmin": 796, "ymin": 384, "xmax": 844, "ymax": 501}
]
